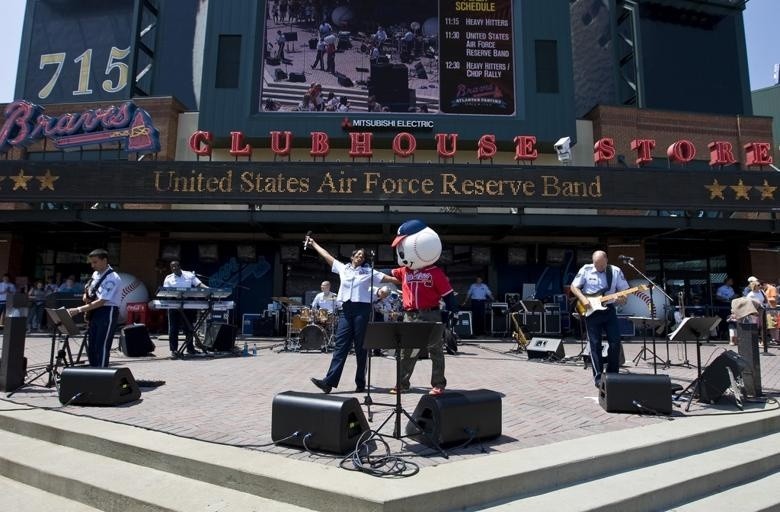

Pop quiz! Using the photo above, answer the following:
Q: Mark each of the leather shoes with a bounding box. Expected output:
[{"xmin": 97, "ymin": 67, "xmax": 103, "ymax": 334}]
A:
[
  {"xmin": 354, "ymin": 382, "xmax": 365, "ymax": 393},
  {"xmin": 311, "ymin": 377, "xmax": 332, "ymax": 394}
]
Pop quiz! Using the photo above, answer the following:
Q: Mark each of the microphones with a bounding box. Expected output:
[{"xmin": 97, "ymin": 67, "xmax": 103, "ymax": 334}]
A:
[
  {"xmin": 618, "ymin": 254, "xmax": 634, "ymax": 260},
  {"xmin": 303, "ymin": 230, "xmax": 313, "ymax": 250},
  {"xmin": 370, "ymin": 248, "xmax": 376, "ymax": 256}
]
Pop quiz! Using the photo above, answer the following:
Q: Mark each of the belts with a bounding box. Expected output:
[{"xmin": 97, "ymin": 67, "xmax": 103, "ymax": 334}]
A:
[{"xmin": 401, "ymin": 306, "xmax": 442, "ymax": 314}]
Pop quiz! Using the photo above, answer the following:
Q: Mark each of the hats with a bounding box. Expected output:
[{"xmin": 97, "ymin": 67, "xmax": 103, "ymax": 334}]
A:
[
  {"xmin": 389, "ymin": 218, "xmax": 427, "ymax": 247},
  {"xmin": 748, "ymin": 276, "xmax": 758, "ymax": 281}
]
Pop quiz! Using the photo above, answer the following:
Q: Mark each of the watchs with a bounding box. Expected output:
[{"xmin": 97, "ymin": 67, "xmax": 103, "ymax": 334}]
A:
[{"xmin": 77, "ymin": 306, "xmax": 82, "ymax": 313}]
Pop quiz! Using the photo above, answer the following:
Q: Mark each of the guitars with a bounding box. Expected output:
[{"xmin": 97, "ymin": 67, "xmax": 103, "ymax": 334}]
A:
[{"xmin": 576, "ymin": 284, "xmax": 655, "ymax": 317}]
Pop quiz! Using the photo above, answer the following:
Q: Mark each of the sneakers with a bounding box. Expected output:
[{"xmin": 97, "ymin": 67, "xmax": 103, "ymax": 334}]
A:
[
  {"xmin": 193, "ymin": 349, "xmax": 201, "ymax": 355},
  {"xmin": 428, "ymin": 385, "xmax": 446, "ymax": 395},
  {"xmin": 389, "ymin": 386, "xmax": 411, "ymax": 394},
  {"xmin": 171, "ymin": 351, "xmax": 178, "ymax": 359}
]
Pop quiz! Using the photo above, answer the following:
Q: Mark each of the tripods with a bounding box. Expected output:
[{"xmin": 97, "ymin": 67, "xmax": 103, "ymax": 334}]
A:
[
  {"xmin": 7, "ymin": 308, "xmax": 81, "ymax": 402},
  {"xmin": 354, "ymin": 322, "xmax": 449, "ymax": 458},
  {"xmin": 670, "ymin": 317, "xmax": 743, "ymax": 411}
]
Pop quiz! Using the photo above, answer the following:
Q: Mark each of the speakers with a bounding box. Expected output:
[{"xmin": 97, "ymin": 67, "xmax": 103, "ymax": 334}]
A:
[
  {"xmin": 737, "ymin": 323, "xmax": 763, "ymax": 398},
  {"xmin": 696, "ymin": 347, "xmax": 746, "ymax": 405},
  {"xmin": 206, "ymin": 322, "xmax": 238, "ymax": 354},
  {"xmin": 405, "ymin": 388, "xmax": 506, "ymax": 450},
  {"xmin": 58, "ymin": 365, "xmax": 141, "ymax": 408},
  {"xmin": 1, "ymin": 317, "xmax": 26, "ymax": 392},
  {"xmin": 598, "ymin": 370, "xmax": 673, "ymax": 415},
  {"xmin": 271, "ymin": 389, "xmax": 372, "ymax": 456},
  {"xmin": 119, "ymin": 325, "xmax": 154, "ymax": 358},
  {"xmin": 526, "ymin": 335, "xmax": 566, "ymax": 362}
]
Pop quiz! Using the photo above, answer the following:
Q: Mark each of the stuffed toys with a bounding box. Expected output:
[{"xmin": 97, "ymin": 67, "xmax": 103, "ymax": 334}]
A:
[{"xmin": 376, "ymin": 220, "xmax": 458, "ymax": 395}]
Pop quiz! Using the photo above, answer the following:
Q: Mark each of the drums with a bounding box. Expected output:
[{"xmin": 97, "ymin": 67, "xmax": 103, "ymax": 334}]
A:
[
  {"xmin": 298, "ymin": 306, "xmax": 312, "ymax": 321},
  {"xmin": 298, "ymin": 323, "xmax": 328, "ymax": 351},
  {"xmin": 310, "ymin": 309, "xmax": 320, "ymax": 322},
  {"xmin": 313, "ymin": 308, "xmax": 329, "ymax": 322}
]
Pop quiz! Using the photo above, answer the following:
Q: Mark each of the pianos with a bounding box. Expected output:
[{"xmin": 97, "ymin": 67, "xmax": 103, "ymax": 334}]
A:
[{"xmin": 148, "ymin": 286, "xmax": 236, "ymax": 311}]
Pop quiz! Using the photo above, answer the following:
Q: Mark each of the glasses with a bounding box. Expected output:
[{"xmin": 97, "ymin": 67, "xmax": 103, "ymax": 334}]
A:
[{"xmin": 757, "ymin": 285, "xmax": 760, "ymax": 286}]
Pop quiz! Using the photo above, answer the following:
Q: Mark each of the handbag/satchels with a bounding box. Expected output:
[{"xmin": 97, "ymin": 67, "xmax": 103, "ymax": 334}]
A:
[{"xmin": 760, "ymin": 290, "xmax": 768, "ymax": 307}]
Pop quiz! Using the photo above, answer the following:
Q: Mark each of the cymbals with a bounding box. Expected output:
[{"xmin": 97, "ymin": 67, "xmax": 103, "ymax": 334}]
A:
[
  {"xmin": 285, "ymin": 301, "xmax": 303, "ymax": 307},
  {"xmin": 271, "ymin": 296, "xmax": 291, "ymax": 303},
  {"xmin": 323, "ymin": 293, "xmax": 338, "ymax": 300}
]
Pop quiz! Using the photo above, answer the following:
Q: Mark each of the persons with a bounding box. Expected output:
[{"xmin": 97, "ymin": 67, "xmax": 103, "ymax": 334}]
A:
[
  {"xmin": 164, "ymin": 262, "xmax": 210, "ymax": 356},
  {"xmin": 1, "ymin": 274, "xmax": 17, "ymax": 324},
  {"xmin": 464, "ymin": 277, "xmax": 498, "ymax": 337},
  {"xmin": 747, "ymin": 281, "xmax": 769, "ymax": 345},
  {"xmin": 320, "ymin": 20, "xmax": 332, "ymax": 35},
  {"xmin": 296, "ymin": 0, "xmax": 303, "ymax": 24},
  {"xmin": 370, "ymin": 45, "xmax": 379, "ymax": 65},
  {"xmin": 337, "ymin": 96, "xmax": 350, "ymax": 111},
  {"xmin": 325, "ymin": 93, "xmax": 339, "ymax": 111},
  {"xmin": 68, "ymin": 250, "xmax": 123, "ymax": 368},
  {"xmin": 325, "ymin": 31, "xmax": 336, "ymax": 74},
  {"xmin": 312, "ymin": 281, "xmax": 343, "ymax": 314},
  {"xmin": 311, "ymin": 37, "xmax": 326, "ymax": 70},
  {"xmin": 716, "ymin": 278, "xmax": 736, "ymax": 340},
  {"xmin": 303, "ymin": 236, "xmax": 402, "ymax": 393},
  {"xmin": 288, "ymin": 0, "xmax": 295, "ymax": 23},
  {"xmin": 570, "ymin": 251, "xmax": 630, "ymax": 388},
  {"xmin": 278, "ymin": 0, "xmax": 287, "ymax": 22},
  {"xmin": 371, "ymin": 286, "xmax": 391, "ymax": 321},
  {"xmin": 760, "ymin": 282, "xmax": 777, "ymax": 344},
  {"xmin": 276, "ymin": 31, "xmax": 284, "ymax": 60},
  {"xmin": 305, "ymin": 0, "xmax": 312, "ymax": 24},
  {"xmin": 400, "ymin": 27, "xmax": 414, "ymax": 54},
  {"xmin": 743, "ymin": 275, "xmax": 758, "ymax": 297},
  {"xmin": 375, "ymin": 26, "xmax": 387, "ymax": 48},
  {"xmin": 18, "ymin": 276, "xmax": 83, "ymax": 334},
  {"xmin": 305, "ymin": 83, "xmax": 315, "ymax": 95},
  {"xmin": 271, "ymin": 2, "xmax": 278, "ymax": 24},
  {"xmin": 298, "ymin": 95, "xmax": 314, "ymax": 111},
  {"xmin": 311, "ymin": 84, "xmax": 325, "ymax": 111}
]
